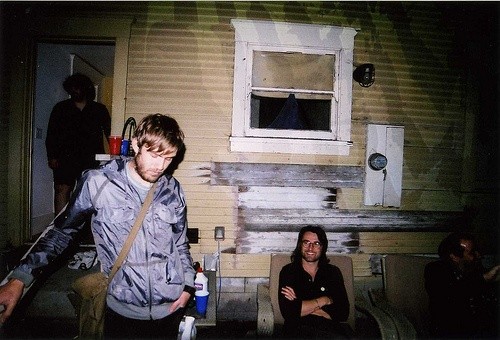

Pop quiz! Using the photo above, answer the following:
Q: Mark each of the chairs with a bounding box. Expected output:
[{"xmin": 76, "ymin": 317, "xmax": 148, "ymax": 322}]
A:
[
  {"xmin": 256, "ymin": 254, "xmax": 399, "ymax": 340},
  {"xmin": 368, "ymin": 255, "xmax": 436, "ymax": 340}
]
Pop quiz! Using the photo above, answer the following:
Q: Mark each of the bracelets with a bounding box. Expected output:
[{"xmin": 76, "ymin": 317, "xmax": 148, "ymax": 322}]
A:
[{"xmin": 315, "ymin": 299, "xmax": 321, "ymax": 309}]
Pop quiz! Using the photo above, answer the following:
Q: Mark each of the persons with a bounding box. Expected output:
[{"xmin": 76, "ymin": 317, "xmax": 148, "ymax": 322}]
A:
[
  {"xmin": 45, "ymin": 73, "xmax": 111, "ymax": 217},
  {"xmin": 0, "ymin": 112, "xmax": 195, "ymax": 340},
  {"xmin": 278, "ymin": 225, "xmax": 354, "ymax": 340},
  {"xmin": 424, "ymin": 232, "xmax": 500, "ymax": 340}
]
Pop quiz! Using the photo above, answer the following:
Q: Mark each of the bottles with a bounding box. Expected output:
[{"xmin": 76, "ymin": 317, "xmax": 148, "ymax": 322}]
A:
[{"xmin": 194, "ymin": 268, "xmax": 209, "ymax": 300}]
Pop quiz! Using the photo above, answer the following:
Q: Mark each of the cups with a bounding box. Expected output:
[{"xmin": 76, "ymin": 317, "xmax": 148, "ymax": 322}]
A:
[
  {"xmin": 194, "ymin": 290, "xmax": 210, "ymax": 313},
  {"xmin": 109, "ymin": 136, "xmax": 129, "ymax": 157}
]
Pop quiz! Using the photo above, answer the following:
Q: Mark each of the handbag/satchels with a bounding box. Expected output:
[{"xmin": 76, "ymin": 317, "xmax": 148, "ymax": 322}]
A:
[{"xmin": 71, "ymin": 271, "xmax": 109, "ymax": 340}]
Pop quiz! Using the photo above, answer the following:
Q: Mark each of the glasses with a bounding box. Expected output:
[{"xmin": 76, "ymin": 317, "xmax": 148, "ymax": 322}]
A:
[{"xmin": 302, "ymin": 240, "xmax": 321, "ymax": 248}]
